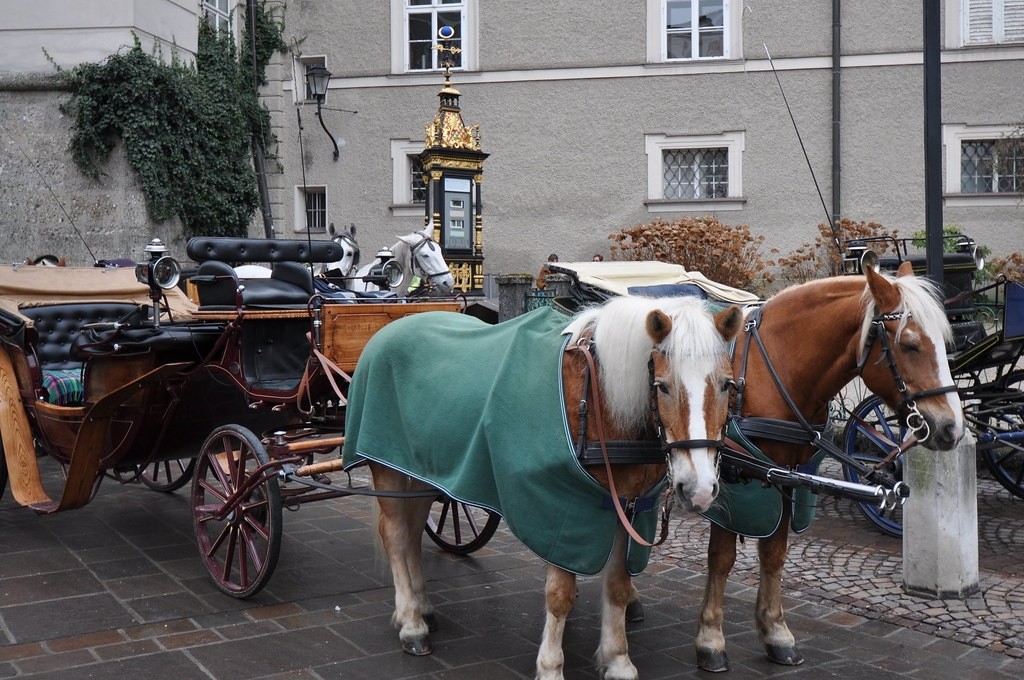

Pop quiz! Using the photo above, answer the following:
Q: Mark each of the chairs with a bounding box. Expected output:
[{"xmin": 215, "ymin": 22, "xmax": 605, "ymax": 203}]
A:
[{"xmin": 186, "ymin": 235, "xmax": 344, "ymax": 309}]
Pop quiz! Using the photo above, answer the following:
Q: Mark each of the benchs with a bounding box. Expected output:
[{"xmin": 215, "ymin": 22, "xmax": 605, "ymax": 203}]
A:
[
  {"xmin": 19, "ymin": 303, "xmax": 155, "ymax": 399},
  {"xmin": 846, "ymin": 234, "xmax": 977, "ymax": 276}
]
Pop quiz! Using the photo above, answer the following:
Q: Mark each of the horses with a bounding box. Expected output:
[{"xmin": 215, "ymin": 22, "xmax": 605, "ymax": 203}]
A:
[
  {"xmin": 232, "ymin": 222, "xmax": 360, "ymax": 286},
  {"xmin": 366, "ymin": 295, "xmax": 743, "ymax": 680},
  {"xmin": 623, "ymin": 260, "xmax": 967, "ymax": 672},
  {"xmin": 308, "ymin": 217, "xmax": 456, "ymax": 304}
]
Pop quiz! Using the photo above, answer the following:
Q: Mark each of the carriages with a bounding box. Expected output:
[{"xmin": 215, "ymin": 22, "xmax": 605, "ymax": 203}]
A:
[{"xmin": 0, "ymin": 237, "xmax": 969, "ymax": 680}]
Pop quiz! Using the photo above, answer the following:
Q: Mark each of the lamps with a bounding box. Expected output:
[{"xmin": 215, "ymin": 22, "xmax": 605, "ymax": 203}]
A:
[{"xmin": 304, "ymin": 62, "xmax": 358, "ymax": 161}]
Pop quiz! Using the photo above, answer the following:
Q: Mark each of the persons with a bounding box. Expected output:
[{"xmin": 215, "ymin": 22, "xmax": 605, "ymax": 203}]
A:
[
  {"xmin": 536, "ymin": 254, "xmax": 558, "ymax": 290},
  {"xmin": 592, "ymin": 255, "xmax": 603, "ymax": 262}
]
